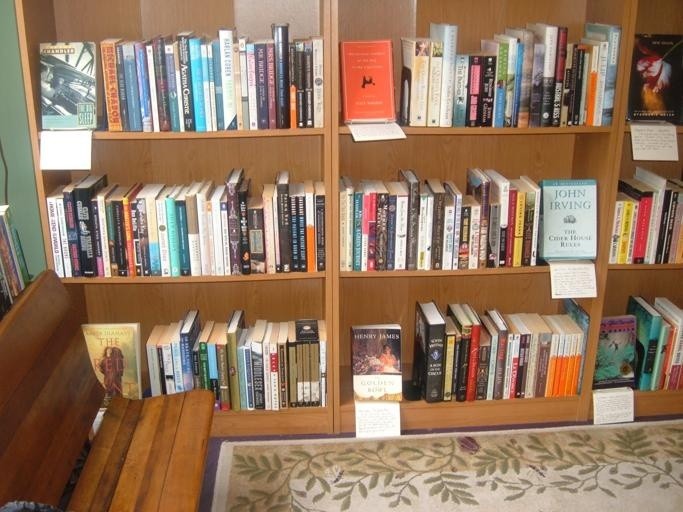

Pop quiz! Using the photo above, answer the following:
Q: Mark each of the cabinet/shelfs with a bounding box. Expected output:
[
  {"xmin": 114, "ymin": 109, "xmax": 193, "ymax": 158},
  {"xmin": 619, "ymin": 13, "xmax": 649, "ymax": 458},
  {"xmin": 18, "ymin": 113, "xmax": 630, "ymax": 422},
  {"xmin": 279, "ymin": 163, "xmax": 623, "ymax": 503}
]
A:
[
  {"xmin": 15, "ymin": 0, "xmax": 333, "ymax": 438},
  {"xmin": 333, "ymin": 0, "xmax": 633, "ymax": 439},
  {"xmin": 585, "ymin": 1, "xmax": 683, "ymax": 424}
]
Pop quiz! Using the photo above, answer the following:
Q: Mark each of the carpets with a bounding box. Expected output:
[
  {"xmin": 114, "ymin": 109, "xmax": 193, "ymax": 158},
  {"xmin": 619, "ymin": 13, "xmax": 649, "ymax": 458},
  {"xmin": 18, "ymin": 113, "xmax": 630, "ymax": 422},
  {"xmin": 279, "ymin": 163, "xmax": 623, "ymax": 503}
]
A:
[{"xmin": 210, "ymin": 418, "xmax": 683, "ymax": 512}]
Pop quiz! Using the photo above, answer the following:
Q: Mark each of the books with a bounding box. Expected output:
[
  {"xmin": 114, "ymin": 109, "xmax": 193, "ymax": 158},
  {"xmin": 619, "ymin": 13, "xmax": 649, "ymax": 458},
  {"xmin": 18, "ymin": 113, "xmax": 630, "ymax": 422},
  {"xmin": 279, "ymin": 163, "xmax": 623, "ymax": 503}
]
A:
[
  {"xmin": 591, "ymin": 295, "xmax": 682, "ymax": 412},
  {"xmin": 609, "ymin": 166, "xmax": 682, "ymax": 263},
  {"xmin": 37, "ymin": 23, "xmax": 325, "ymax": 131},
  {"xmin": 626, "ymin": 33, "xmax": 682, "ymax": 125},
  {"xmin": 48, "ymin": 167, "xmax": 325, "ymax": 278},
  {"xmin": 351, "ymin": 302, "xmax": 590, "ymax": 411},
  {"xmin": 338, "ymin": 166, "xmax": 600, "ymax": 299},
  {"xmin": 0, "ymin": 202, "xmax": 33, "ymax": 317},
  {"xmin": 338, "ymin": 20, "xmax": 619, "ymax": 126},
  {"xmin": 81, "ymin": 309, "xmax": 328, "ymax": 411}
]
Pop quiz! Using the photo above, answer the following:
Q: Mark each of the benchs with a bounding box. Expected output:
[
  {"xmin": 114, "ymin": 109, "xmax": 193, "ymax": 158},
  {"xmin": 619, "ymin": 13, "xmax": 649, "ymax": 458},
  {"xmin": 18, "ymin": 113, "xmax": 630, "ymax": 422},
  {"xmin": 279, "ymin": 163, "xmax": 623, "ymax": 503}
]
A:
[{"xmin": 0, "ymin": 271, "xmax": 215, "ymax": 512}]
